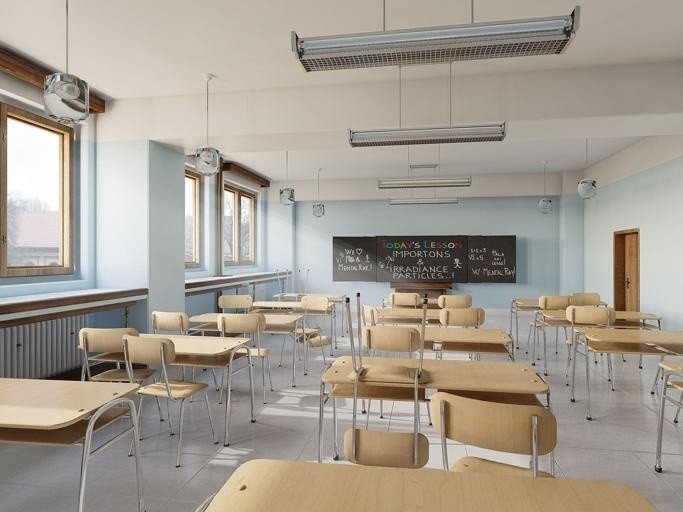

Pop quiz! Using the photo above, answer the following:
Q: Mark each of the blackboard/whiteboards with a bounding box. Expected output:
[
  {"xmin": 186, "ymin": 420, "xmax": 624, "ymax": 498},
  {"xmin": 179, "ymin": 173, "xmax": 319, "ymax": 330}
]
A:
[{"xmin": 332, "ymin": 235, "xmax": 517, "ymax": 283}]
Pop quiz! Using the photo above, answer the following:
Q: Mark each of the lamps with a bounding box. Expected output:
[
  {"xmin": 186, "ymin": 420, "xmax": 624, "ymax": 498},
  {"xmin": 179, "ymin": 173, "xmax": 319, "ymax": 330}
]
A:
[
  {"xmin": 577, "ymin": 136, "xmax": 599, "ymax": 200},
  {"xmin": 347, "ymin": 61, "xmax": 507, "ymax": 148},
  {"xmin": 377, "ymin": 143, "xmax": 472, "ymax": 190},
  {"xmin": 538, "ymin": 160, "xmax": 553, "ymax": 215},
  {"xmin": 194, "ymin": 75, "xmax": 222, "ymax": 177},
  {"xmin": 312, "ymin": 168, "xmax": 325, "ymax": 218},
  {"xmin": 289, "ymin": 0, "xmax": 582, "ymax": 73},
  {"xmin": 278, "ymin": 150, "xmax": 296, "ymax": 208},
  {"xmin": 42, "ymin": 0, "xmax": 91, "ymax": 124},
  {"xmin": 387, "ymin": 164, "xmax": 461, "ymax": 206}
]
